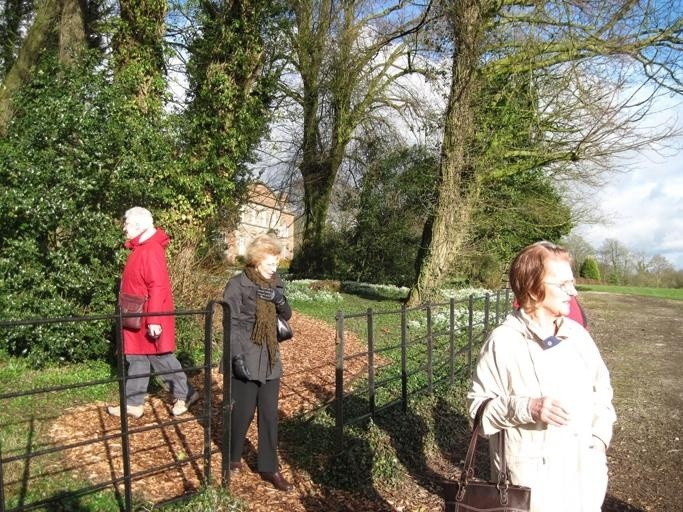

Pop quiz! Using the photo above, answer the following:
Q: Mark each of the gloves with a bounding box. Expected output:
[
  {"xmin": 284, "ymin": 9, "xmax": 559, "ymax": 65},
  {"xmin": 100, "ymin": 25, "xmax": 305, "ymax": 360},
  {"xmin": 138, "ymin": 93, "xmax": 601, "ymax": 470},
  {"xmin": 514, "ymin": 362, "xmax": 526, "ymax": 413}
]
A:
[
  {"xmin": 255, "ymin": 286, "xmax": 281, "ymax": 304},
  {"xmin": 230, "ymin": 353, "xmax": 252, "ymax": 383}
]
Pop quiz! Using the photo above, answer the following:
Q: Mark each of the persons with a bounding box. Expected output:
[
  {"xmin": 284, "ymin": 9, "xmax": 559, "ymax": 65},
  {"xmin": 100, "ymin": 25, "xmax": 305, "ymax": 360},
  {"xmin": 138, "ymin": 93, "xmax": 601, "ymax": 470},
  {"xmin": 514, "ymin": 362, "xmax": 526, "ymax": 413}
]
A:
[
  {"xmin": 222, "ymin": 235, "xmax": 295, "ymax": 493},
  {"xmin": 468, "ymin": 241, "xmax": 617, "ymax": 512},
  {"xmin": 106, "ymin": 206, "xmax": 200, "ymax": 417}
]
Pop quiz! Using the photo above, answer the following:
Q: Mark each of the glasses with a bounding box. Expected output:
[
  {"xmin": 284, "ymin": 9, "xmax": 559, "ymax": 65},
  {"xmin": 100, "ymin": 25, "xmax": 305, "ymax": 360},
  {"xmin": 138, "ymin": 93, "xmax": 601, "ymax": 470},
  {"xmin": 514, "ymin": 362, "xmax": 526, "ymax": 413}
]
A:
[{"xmin": 542, "ymin": 278, "xmax": 574, "ymax": 291}]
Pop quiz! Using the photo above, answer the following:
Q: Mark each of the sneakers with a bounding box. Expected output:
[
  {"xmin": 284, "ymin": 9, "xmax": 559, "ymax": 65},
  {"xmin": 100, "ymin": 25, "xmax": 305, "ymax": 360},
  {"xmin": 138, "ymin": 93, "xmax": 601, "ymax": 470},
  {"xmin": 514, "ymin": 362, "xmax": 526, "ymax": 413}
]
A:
[
  {"xmin": 106, "ymin": 402, "xmax": 145, "ymax": 419},
  {"xmin": 170, "ymin": 390, "xmax": 201, "ymax": 417}
]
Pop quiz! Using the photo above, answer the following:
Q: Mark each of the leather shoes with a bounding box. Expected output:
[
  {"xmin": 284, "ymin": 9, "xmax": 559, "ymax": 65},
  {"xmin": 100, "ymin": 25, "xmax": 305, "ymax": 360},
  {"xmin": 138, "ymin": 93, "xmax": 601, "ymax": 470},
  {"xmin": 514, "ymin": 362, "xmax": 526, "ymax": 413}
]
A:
[
  {"xmin": 259, "ymin": 469, "xmax": 293, "ymax": 491},
  {"xmin": 229, "ymin": 460, "xmax": 242, "ymax": 472}
]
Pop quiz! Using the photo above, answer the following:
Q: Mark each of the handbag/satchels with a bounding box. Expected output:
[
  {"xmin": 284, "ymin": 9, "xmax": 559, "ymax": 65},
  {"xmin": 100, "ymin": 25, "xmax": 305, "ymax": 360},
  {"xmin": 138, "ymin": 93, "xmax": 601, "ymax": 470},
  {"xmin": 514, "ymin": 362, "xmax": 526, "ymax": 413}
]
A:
[
  {"xmin": 116, "ymin": 292, "xmax": 147, "ymax": 331},
  {"xmin": 439, "ymin": 396, "xmax": 533, "ymax": 512},
  {"xmin": 274, "ymin": 309, "xmax": 294, "ymax": 343}
]
